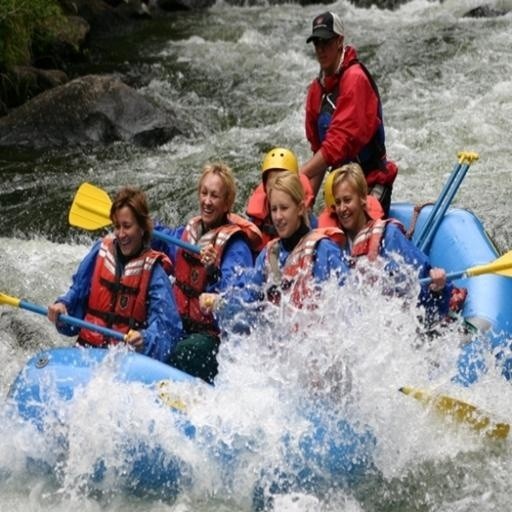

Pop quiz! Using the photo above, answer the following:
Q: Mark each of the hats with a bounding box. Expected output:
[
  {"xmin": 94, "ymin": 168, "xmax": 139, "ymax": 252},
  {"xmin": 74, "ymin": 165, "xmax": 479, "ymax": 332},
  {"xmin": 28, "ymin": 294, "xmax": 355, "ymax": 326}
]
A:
[{"xmin": 306, "ymin": 12, "xmax": 343, "ymax": 43}]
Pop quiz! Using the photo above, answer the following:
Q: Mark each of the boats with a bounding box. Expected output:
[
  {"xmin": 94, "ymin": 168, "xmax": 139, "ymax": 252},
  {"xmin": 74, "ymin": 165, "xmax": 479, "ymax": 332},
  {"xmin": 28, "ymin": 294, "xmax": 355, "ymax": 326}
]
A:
[{"xmin": 5, "ymin": 203, "xmax": 512, "ymax": 512}]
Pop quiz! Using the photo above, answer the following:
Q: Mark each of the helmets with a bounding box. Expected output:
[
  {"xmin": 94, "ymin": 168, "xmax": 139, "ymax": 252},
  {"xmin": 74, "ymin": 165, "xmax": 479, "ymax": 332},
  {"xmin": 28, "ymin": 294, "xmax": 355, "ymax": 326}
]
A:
[
  {"xmin": 262, "ymin": 148, "xmax": 299, "ymax": 192},
  {"xmin": 324, "ymin": 170, "xmax": 337, "ymax": 208}
]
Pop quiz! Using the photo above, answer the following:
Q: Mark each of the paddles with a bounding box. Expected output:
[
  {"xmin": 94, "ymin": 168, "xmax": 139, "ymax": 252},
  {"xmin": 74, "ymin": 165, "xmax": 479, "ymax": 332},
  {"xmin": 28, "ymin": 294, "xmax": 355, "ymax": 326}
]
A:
[
  {"xmin": 399, "ymin": 386, "xmax": 510, "ymax": 445},
  {"xmin": 386, "ymin": 249, "xmax": 512, "ymax": 292},
  {"xmin": 69, "ymin": 183, "xmax": 205, "ymax": 256}
]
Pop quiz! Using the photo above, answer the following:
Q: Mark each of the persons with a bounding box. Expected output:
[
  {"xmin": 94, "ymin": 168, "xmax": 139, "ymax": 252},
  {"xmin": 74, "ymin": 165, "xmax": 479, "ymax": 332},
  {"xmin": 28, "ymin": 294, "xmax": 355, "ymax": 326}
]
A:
[
  {"xmin": 332, "ymin": 161, "xmax": 453, "ymax": 326},
  {"xmin": 48, "ymin": 188, "xmax": 183, "ymax": 362},
  {"xmin": 218, "ymin": 172, "xmax": 352, "ymax": 337},
  {"xmin": 152, "ymin": 163, "xmax": 254, "ymax": 380},
  {"xmin": 246, "ymin": 148, "xmax": 318, "ymax": 242},
  {"xmin": 318, "ymin": 168, "xmax": 385, "ymax": 228},
  {"xmin": 301, "ymin": 12, "xmax": 398, "ymax": 220}
]
